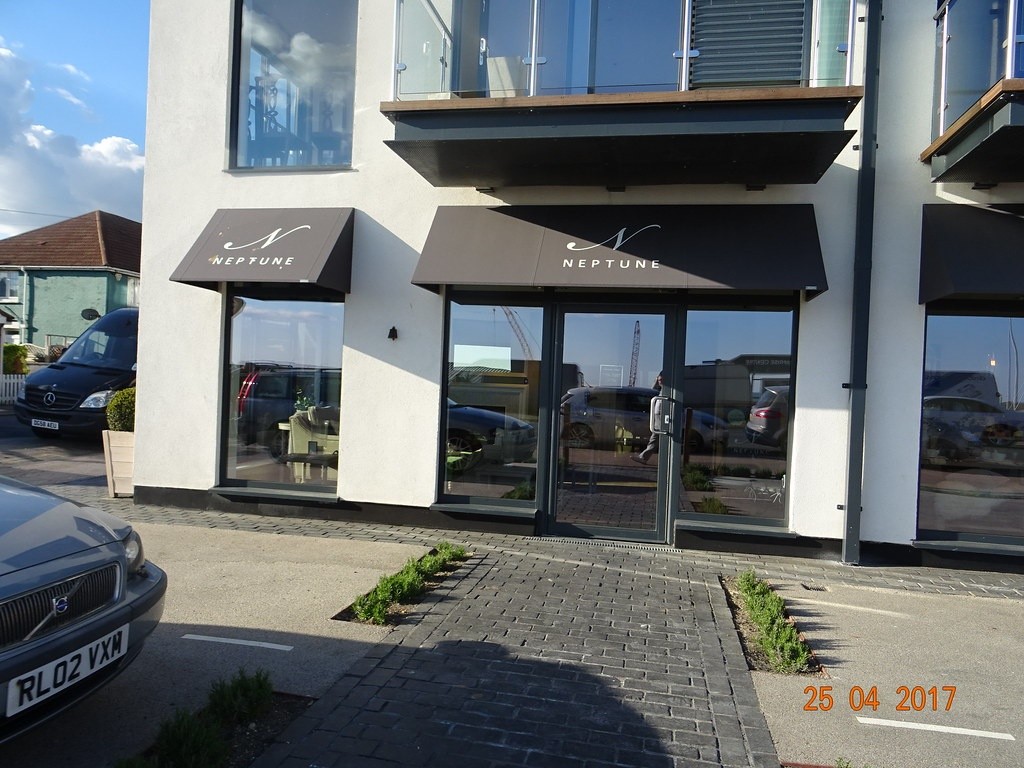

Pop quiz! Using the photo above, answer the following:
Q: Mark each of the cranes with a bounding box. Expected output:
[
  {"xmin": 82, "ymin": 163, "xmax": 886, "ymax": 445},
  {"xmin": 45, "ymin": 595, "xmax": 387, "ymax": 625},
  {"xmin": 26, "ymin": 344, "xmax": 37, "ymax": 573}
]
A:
[
  {"xmin": 628, "ymin": 319, "xmax": 639, "ymax": 387},
  {"xmin": 493, "ymin": 306, "xmax": 541, "ymax": 361}
]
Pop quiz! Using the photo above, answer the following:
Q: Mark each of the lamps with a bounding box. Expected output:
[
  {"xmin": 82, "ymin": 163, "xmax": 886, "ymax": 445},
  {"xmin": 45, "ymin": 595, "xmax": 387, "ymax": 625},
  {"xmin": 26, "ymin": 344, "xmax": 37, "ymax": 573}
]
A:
[{"xmin": 386, "ymin": 326, "xmax": 399, "ymax": 340}]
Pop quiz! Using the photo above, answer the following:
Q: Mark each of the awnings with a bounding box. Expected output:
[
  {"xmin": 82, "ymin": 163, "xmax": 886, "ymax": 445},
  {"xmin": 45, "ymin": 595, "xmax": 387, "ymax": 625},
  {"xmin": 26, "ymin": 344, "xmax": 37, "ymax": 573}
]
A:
[
  {"xmin": 169, "ymin": 210, "xmax": 356, "ymax": 302},
  {"xmin": 410, "ymin": 202, "xmax": 830, "ymax": 313},
  {"xmin": 918, "ymin": 202, "xmax": 1023, "ymax": 307}
]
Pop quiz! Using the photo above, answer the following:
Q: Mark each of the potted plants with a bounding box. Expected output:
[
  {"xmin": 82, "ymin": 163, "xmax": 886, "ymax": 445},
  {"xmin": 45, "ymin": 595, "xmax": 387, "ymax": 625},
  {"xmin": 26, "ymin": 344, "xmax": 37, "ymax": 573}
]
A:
[{"xmin": 102, "ymin": 387, "xmax": 134, "ymax": 497}]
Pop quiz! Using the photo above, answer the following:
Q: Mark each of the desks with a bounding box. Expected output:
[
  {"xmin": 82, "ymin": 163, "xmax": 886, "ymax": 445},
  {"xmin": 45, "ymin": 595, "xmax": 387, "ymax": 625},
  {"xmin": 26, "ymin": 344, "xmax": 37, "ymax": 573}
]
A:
[{"xmin": 286, "ymin": 452, "xmax": 337, "ymax": 482}]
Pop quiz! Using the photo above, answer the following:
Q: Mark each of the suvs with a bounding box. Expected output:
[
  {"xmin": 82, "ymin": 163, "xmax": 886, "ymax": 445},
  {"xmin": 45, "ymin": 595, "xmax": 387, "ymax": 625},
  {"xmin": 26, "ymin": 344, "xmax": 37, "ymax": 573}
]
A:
[{"xmin": 234, "ymin": 364, "xmax": 342, "ymax": 464}]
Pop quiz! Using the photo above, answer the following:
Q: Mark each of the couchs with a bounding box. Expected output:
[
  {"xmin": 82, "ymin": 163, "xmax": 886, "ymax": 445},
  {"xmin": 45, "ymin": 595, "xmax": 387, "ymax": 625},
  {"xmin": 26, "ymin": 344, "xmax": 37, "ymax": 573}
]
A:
[{"xmin": 288, "ymin": 405, "xmax": 339, "ymax": 483}]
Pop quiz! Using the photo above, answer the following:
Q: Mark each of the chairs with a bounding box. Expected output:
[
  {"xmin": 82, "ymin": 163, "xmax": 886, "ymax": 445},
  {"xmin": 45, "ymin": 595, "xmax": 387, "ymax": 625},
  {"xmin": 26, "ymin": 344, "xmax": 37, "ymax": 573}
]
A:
[{"xmin": 247, "ymin": 75, "xmax": 350, "ymax": 164}]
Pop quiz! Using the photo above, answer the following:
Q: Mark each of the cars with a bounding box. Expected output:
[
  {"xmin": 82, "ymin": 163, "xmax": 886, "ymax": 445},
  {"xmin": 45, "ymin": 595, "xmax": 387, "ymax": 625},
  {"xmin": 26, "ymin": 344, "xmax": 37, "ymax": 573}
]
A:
[
  {"xmin": 559, "ymin": 386, "xmax": 729, "ymax": 454},
  {"xmin": 15, "ymin": 306, "xmax": 138, "ymax": 441},
  {"xmin": 0, "ymin": 475, "xmax": 168, "ymax": 746},
  {"xmin": 919, "ymin": 395, "xmax": 1024, "ymax": 464},
  {"xmin": 446, "ymin": 398, "xmax": 537, "ymax": 470},
  {"xmin": 746, "ymin": 384, "xmax": 790, "ymax": 453}
]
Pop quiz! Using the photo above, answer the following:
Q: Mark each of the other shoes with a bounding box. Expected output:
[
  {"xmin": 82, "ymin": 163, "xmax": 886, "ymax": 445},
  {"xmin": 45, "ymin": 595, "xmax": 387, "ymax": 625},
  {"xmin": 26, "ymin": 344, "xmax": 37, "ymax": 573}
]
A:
[{"xmin": 630, "ymin": 456, "xmax": 648, "ymax": 464}]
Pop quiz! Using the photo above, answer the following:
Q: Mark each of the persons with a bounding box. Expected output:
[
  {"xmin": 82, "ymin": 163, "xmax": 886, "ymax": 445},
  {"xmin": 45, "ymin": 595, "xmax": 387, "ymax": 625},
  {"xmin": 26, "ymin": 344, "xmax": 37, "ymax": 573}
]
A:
[{"xmin": 630, "ymin": 368, "xmax": 689, "ymax": 467}]
